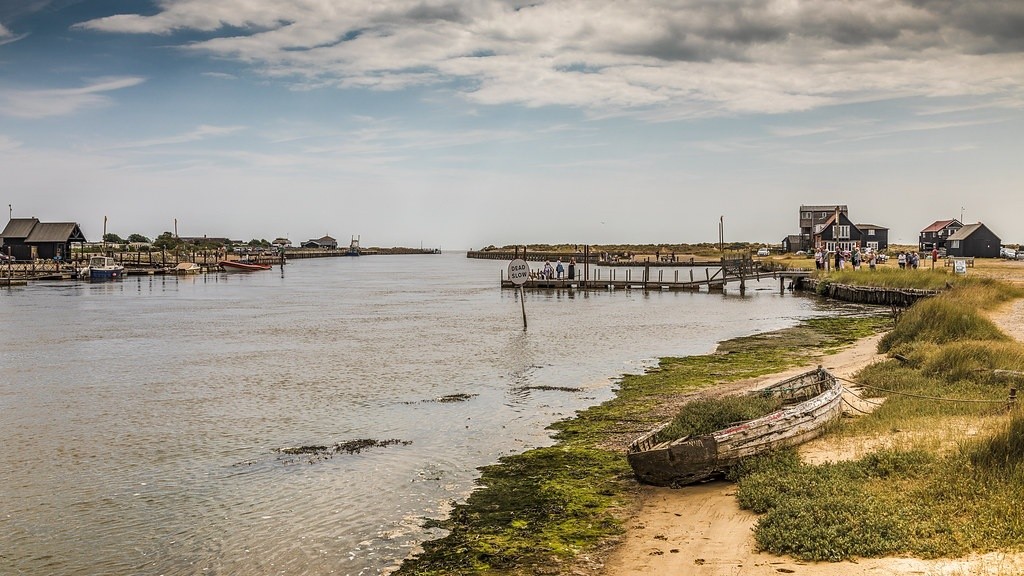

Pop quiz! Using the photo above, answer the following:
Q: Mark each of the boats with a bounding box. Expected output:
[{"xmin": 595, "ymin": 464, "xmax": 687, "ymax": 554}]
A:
[
  {"xmin": 234, "ymin": 246, "xmax": 265, "ymax": 255},
  {"xmin": 170, "ymin": 262, "xmax": 200, "ymax": 275},
  {"xmin": 627, "ymin": 364, "xmax": 847, "ymax": 489},
  {"xmin": 345, "ymin": 247, "xmax": 361, "ymax": 257},
  {"xmin": 87, "ymin": 256, "xmax": 125, "ymax": 280},
  {"xmin": 218, "ymin": 261, "xmax": 272, "ymax": 272}
]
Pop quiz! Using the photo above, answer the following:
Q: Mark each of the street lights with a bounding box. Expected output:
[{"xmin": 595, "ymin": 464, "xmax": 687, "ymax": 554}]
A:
[{"xmin": 961, "ymin": 206, "xmax": 967, "ymax": 224}]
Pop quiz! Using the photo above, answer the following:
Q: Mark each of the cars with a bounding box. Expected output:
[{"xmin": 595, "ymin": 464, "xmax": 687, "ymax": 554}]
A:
[
  {"xmin": 1000, "ymin": 248, "xmax": 1024, "ymax": 260},
  {"xmin": 757, "ymin": 248, "xmax": 770, "ymax": 257}
]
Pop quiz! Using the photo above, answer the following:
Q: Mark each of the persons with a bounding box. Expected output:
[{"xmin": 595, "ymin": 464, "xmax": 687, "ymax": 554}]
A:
[
  {"xmin": 556, "ymin": 256, "xmax": 563, "ymax": 280},
  {"xmin": 814, "ymin": 245, "xmax": 920, "ymax": 271},
  {"xmin": 570, "ymin": 256, "xmax": 576, "ymax": 266},
  {"xmin": 544, "ymin": 260, "xmax": 553, "ymax": 279}
]
